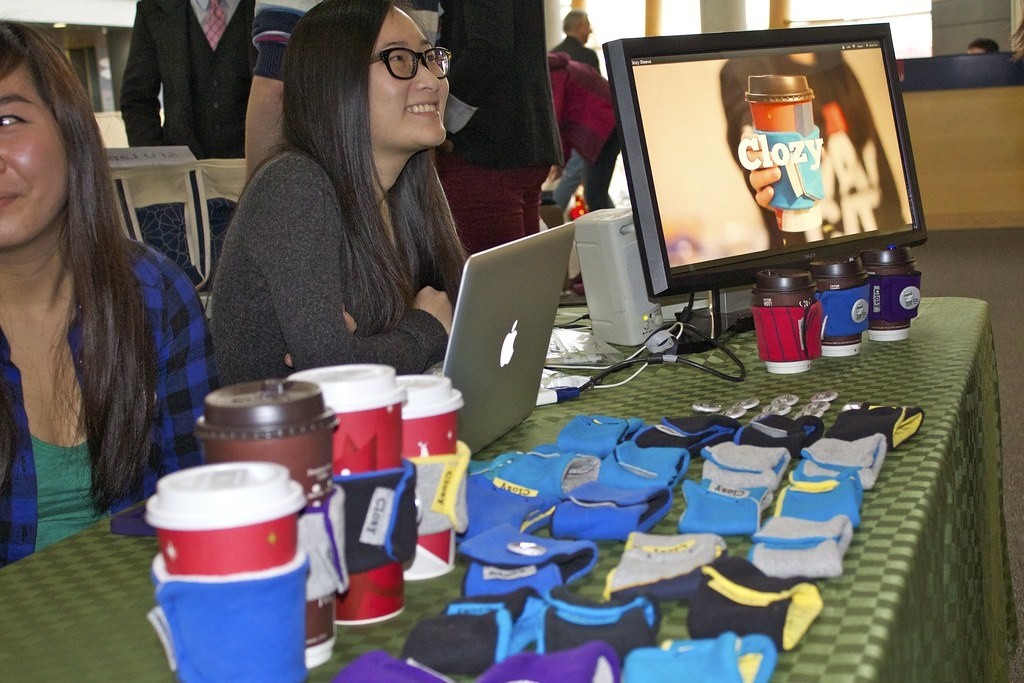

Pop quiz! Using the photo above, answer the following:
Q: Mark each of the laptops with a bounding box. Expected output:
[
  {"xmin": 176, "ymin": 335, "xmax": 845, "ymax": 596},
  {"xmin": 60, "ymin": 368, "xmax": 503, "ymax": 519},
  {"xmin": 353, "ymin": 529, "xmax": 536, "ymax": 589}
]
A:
[{"xmin": 440, "ymin": 222, "xmax": 575, "ymax": 455}]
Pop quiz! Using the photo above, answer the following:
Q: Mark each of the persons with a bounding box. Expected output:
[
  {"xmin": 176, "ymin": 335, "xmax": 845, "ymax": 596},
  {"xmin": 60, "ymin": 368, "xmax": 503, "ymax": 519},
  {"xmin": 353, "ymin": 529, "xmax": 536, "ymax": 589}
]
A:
[
  {"xmin": 433, "ymin": 0, "xmax": 625, "ymax": 264},
  {"xmin": 207, "ymin": 0, "xmax": 470, "ymax": 388},
  {"xmin": 121, "ymin": 0, "xmax": 257, "ymax": 159},
  {"xmin": 0, "ymin": 20, "xmax": 222, "ymax": 569},
  {"xmin": 967, "ymin": 37, "xmax": 999, "ymax": 54},
  {"xmin": 719, "ymin": 50, "xmax": 904, "ymax": 249}
]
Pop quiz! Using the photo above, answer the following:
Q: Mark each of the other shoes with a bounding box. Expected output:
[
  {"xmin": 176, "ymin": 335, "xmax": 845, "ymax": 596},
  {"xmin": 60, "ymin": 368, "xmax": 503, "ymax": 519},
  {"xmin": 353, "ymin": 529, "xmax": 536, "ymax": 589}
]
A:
[{"xmin": 573, "ymin": 283, "xmax": 585, "ymax": 294}]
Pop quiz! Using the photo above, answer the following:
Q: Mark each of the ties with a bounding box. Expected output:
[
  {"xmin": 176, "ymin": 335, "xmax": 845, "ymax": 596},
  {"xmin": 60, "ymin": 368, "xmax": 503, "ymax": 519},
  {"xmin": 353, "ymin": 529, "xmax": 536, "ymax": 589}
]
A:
[{"xmin": 205, "ymin": 0, "xmax": 225, "ymax": 51}]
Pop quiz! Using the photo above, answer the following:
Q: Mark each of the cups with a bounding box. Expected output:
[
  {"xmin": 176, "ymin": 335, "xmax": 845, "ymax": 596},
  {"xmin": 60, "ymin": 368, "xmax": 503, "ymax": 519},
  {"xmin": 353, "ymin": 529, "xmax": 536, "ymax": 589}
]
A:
[
  {"xmin": 810, "ymin": 257, "xmax": 869, "ymax": 356},
  {"xmin": 861, "ymin": 247, "xmax": 916, "ymax": 341},
  {"xmin": 144, "ymin": 463, "xmax": 307, "ymax": 577},
  {"xmin": 397, "ymin": 374, "xmax": 464, "ymax": 581},
  {"xmin": 751, "ymin": 269, "xmax": 816, "ymax": 374},
  {"xmin": 286, "ymin": 365, "xmax": 406, "ymax": 626},
  {"xmin": 196, "ymin": 382, "xmax": 337, "ymax": 669},
  {"xmin": 745, "ymin": 75, "xmax": 823, "ymax": 232}
]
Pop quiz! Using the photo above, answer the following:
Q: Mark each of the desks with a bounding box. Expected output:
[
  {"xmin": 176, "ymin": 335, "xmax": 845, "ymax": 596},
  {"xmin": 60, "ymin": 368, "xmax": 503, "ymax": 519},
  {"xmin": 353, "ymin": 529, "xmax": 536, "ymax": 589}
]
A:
[{"xmin": 0, "ymin": 295, "xmax": 1020, "ymax": 683}]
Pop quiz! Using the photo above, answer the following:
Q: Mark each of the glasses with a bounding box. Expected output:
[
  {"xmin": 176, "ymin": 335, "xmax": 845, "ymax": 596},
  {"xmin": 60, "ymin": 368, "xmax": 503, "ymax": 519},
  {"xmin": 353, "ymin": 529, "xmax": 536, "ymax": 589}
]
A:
[{"xmin": 370, "ymin": 47, "xmax": 452, "ymax": 79}]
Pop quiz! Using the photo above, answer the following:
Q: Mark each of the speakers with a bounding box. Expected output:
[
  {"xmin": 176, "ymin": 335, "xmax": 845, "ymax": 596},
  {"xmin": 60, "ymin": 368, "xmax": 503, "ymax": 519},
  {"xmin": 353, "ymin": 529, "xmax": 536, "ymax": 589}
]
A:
[{"xmin": 573, "ymin": 207, "xmax": 663, "ymax": 345}]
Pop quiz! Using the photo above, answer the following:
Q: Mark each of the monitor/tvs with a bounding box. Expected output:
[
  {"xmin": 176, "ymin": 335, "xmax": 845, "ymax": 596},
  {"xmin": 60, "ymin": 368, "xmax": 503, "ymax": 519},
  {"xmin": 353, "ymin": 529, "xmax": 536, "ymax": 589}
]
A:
[{"xmin": 602, "ymin": 21, "xmax": 929, "ymax": 335}]
